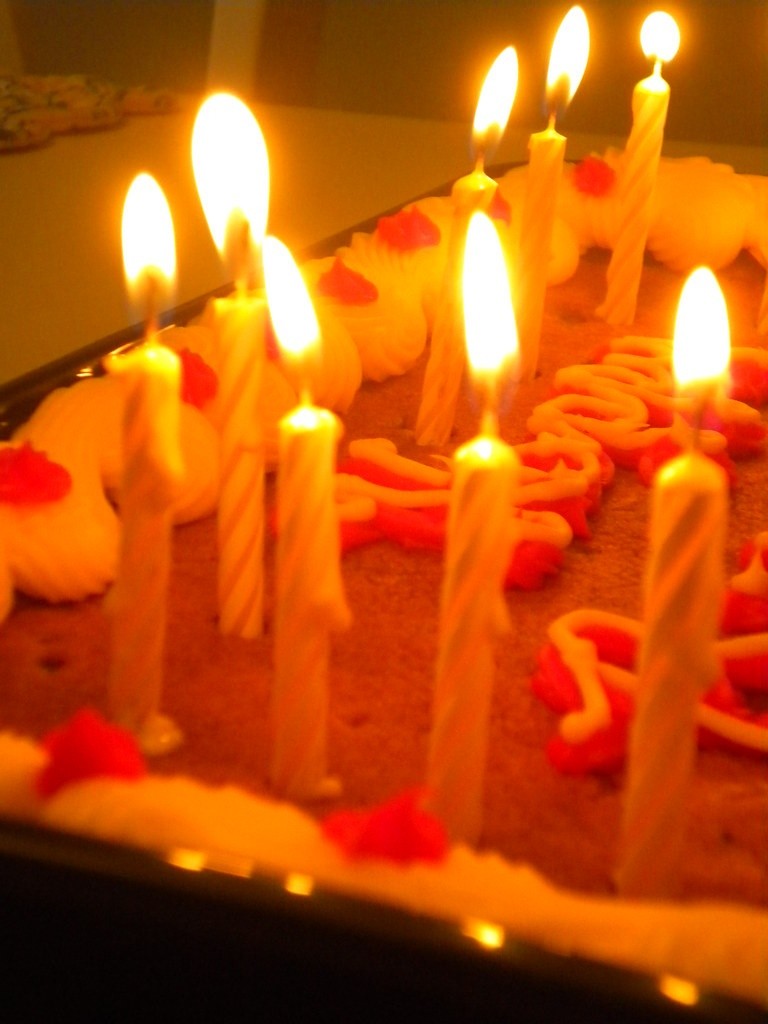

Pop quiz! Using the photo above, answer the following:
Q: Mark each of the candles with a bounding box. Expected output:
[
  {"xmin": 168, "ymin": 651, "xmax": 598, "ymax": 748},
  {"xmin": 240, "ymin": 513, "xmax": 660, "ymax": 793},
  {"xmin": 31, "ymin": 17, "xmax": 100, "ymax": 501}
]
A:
[
  {"xmin": 595, "ymin": 10, "xmax": 681, "ymax": 326},
  {"xmin": 426, "ymin": 208, "xmax": 520, "ymax": 843},
  {"xmin": 257, "ymin": 229, "xmax": 354, "ymax": 803},
  {"xmin": 190, "ymin": 87, "xmax": 275, "ymax": 642},
  {"xmin": 111, "ymin": 168, "xmax": 190, "ymax": 745},
  {"xmin": 414, "ymin": 43, "xmax": 525, "ymax": 451},
  {"xmin": 512, "ymin": 3, "xmax": 594, "ymax": 382},
  {"xmin": 608, "ymin": 263, "xmax": 735, "ymax": 901}
]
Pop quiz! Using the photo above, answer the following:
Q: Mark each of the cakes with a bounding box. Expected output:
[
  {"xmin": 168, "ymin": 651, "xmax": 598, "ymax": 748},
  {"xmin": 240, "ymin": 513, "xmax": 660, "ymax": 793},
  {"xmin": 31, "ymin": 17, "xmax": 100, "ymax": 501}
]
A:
[{"xmin": 0, "ymin": 150, "xmax": 768, "ymax": 1010}]
